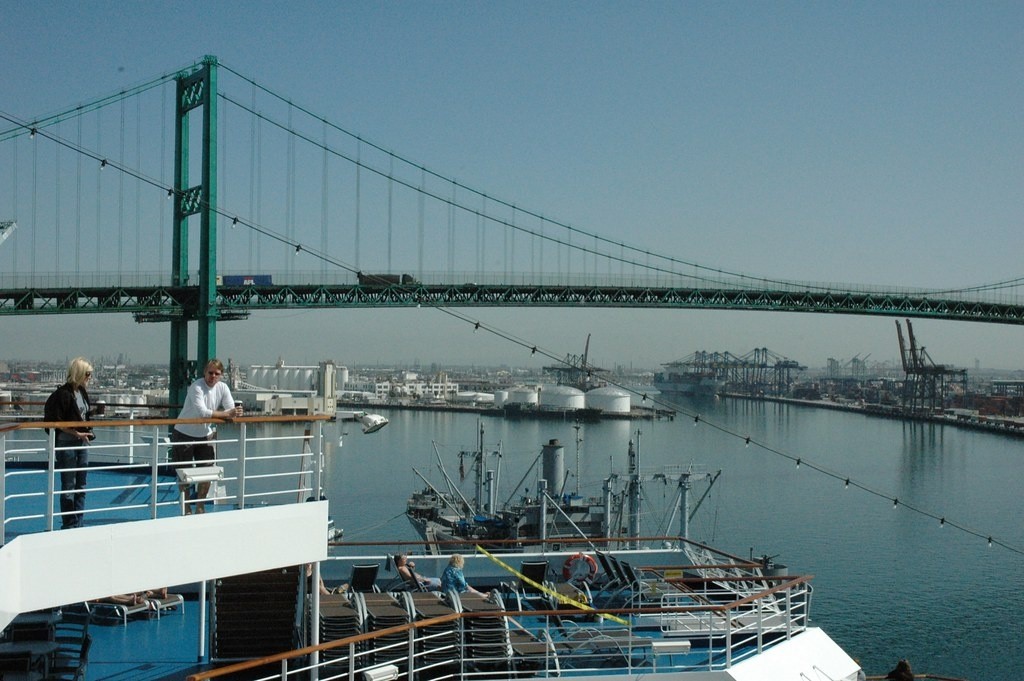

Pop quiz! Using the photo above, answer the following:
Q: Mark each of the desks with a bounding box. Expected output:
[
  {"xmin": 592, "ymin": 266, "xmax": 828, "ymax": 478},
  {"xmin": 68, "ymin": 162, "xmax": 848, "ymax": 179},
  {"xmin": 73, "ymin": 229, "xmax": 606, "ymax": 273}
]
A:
[{"xmin": 0, "ymin": 640, "xmax": 58, "ymax": 679}]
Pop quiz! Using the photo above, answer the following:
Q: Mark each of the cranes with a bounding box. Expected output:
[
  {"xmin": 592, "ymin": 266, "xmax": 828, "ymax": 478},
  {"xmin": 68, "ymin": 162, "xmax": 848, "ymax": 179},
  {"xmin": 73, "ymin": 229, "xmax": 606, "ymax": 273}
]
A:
[
  {"xmin": 661, "ymin": 346, "xmax": 807, "ymax": 399},
  {"xmin": 894, "ymin": 319, "xmax": 970, "ymax": 414}
]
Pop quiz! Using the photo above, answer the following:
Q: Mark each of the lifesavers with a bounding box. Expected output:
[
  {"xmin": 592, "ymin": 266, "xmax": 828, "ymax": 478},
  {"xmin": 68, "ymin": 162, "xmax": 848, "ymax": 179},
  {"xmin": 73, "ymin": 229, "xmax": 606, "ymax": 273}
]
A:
[{"xmin": 563, "ymin": 553, "xmax": 597, "ymax": 581}]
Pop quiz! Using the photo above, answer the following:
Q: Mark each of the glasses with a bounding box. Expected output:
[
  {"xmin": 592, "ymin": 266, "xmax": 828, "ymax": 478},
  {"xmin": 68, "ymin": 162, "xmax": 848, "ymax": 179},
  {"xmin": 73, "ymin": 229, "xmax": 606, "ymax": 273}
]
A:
[
  {"xmin": 206, "ymin": 370, "xmax": 221, "ymax": 375},
  {"xmin": 86, "ymin": 372, "xmax": 90, "ymax": 377}
]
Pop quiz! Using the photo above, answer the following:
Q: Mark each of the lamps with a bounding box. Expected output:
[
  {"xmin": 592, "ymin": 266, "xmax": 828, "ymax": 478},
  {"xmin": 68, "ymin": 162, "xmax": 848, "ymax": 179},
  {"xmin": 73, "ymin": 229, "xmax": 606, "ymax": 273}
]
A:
[
  {"xmin": 334, "ymin": 410, "xmax": 389, "ymax": 446},
  {"xmin": 175, "ymin": 467, "xmax": 225, "ymax": 484}
]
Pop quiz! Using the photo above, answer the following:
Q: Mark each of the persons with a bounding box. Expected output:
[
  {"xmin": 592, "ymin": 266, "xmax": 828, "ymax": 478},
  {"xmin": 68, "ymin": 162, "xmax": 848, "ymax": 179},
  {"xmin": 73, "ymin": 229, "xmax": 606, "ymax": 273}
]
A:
[
  {"xmin": 304, "ymin": 562, "xmax": 331, "ymax": 596},
  {"xmin": 394, "ymin": 553, "xmax": 442, "ymax": 592},
  {"xmin": 43, "ymin": 355, "xmax": 106, "ymax": 531},
  {"xmin": 854, "ymin": 658, "xmax": 915, "ymax": 681},
  {"xmin": 170, "ymin": 358, "xmax": 244, "ymax": 515},
  {"xmin": 89, "ymin": 587, "xmax": 168, "ymax": 605},
  {"xmin": 439, "ymin": 554, "xmax": 488, "ymax": 597}
]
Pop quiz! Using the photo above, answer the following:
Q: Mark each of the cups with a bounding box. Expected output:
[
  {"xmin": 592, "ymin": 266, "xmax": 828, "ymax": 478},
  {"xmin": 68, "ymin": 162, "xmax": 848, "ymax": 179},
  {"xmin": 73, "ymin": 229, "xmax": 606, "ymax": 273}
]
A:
[
  {"xmin": 96, "ymin": 401, "xmax": 105, "ymax": 417},
  {"xmin": 235, "ymin": 400, "xmax": 242, "ymax": 407}
]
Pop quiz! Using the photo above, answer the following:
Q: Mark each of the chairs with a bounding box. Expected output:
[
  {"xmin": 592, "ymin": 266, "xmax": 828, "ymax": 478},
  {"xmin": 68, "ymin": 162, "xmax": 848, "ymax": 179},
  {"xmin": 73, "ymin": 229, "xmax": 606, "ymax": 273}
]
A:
[
  {"xmin": 384, "ymin": 553, "xmax": 430, "ymax": 592},
  {"xmin": 0, "ymin": 620, "xmax": 92, "ymax": 681},
  {"xmin": 62, "ymin": 594, "xmax": 184, "ymax": 626},
  {"xmin": 589, "ymin": 549, "xmax": 693, "ymax": 618},
  {"xmin": 307, "ymin": 580, "xmax": 652, "ymax": 681},
  {"xmin": 348, "ymin": 564, "xmax": 381, "ymax": 593},
  {"xmin": 500, "ymin": 560, "xmax": 551, "ymax": 611}
]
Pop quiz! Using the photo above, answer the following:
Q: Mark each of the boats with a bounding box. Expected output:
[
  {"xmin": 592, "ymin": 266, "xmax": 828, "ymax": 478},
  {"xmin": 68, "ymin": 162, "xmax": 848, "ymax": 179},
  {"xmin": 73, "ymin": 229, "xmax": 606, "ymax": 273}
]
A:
[{"xmin": 653, "ymin": 379, "xmax": 726, "ymax": 394}]
